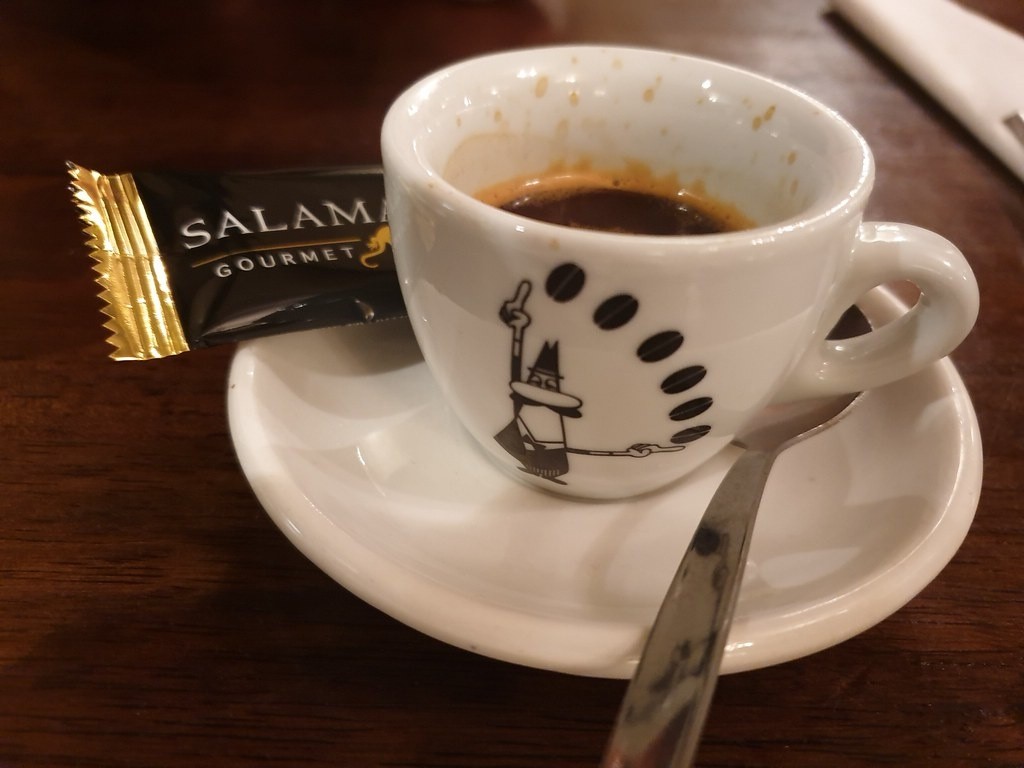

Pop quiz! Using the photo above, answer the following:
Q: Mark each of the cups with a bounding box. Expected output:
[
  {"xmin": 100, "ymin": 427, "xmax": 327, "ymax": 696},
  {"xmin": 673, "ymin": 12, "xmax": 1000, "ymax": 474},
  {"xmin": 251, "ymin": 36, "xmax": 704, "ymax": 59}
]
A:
[{"xmin": 377, "ymin": 42, "xmax": 979, "ymax": 499}]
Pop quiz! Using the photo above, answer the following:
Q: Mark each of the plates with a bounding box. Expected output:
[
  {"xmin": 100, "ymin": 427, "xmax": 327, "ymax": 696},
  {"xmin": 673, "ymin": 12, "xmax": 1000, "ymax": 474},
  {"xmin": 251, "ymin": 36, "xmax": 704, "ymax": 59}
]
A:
[{"xmin": 225, "ymin": 286, "xmax": 981, "ymax": 679}]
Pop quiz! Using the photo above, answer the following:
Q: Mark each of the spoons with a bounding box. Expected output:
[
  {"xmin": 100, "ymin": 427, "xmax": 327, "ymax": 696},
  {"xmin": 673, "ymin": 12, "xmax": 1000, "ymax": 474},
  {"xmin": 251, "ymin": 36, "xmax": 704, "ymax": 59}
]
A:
[{"xmin": 607, "ymin": 299, "xmax": 876, "ymax": 768}]
